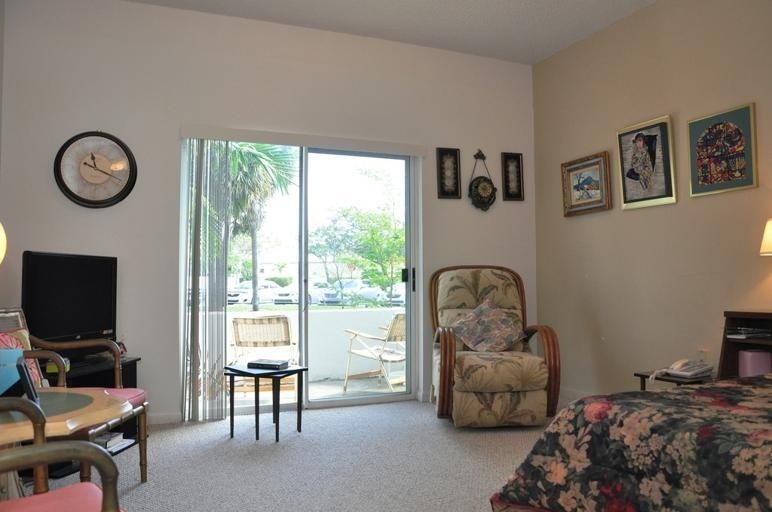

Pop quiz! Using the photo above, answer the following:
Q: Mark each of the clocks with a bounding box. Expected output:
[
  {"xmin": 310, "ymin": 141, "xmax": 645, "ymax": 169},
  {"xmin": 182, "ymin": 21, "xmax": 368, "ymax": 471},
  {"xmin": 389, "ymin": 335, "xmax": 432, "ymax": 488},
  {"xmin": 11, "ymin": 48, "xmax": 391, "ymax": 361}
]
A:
[{"xmin": 53, "ymin": 132, "xmax": 136, "ymax": 207}]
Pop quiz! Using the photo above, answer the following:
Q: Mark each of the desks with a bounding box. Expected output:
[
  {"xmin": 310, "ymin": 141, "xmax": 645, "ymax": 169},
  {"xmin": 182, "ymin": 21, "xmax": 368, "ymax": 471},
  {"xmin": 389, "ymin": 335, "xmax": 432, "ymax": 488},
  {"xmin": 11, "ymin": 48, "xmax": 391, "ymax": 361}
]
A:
[{"xmin": 634, "ymin": 366, "xmax": 712, "ymax": 391}]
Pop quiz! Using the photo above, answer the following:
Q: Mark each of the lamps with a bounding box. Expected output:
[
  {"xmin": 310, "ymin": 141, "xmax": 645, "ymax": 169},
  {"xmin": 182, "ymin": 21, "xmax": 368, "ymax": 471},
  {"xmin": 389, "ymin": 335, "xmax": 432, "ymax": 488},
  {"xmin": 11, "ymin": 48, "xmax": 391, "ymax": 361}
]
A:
[{"xmin": 760, "ymin": 218, "xmax": 772, "ymax": 256}]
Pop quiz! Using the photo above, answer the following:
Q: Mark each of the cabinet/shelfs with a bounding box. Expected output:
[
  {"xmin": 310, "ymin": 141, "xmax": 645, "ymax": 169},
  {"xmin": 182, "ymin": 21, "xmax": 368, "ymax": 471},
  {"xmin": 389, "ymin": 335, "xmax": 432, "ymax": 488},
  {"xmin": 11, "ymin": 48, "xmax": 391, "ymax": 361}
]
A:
[{"xmin": 1, "ymin": 354, "xmax": 150, "ymax": 479}]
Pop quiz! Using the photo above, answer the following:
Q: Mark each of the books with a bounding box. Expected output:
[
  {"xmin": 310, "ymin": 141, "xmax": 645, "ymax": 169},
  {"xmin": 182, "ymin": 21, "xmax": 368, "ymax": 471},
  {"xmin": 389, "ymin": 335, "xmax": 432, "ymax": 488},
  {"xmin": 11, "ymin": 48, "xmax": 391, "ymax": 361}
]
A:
[
  {"xmin": 107, "ymin": 439, "xmax": 135, "ymax": 453},
  {"xmin": 94, "ymin": 431, "xmax": 124, "ymax": 450},
  {"xmin": 247, "ymin": 357, "xmax": 289, "ymax": 371}
]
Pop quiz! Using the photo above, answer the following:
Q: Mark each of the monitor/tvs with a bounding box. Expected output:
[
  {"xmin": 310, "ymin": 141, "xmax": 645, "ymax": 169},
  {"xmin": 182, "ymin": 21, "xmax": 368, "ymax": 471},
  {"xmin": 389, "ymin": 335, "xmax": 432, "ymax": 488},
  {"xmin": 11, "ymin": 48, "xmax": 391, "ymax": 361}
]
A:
[{"xmin": 22, "ymin": 251, "xmax": 117, "ymax": 365}]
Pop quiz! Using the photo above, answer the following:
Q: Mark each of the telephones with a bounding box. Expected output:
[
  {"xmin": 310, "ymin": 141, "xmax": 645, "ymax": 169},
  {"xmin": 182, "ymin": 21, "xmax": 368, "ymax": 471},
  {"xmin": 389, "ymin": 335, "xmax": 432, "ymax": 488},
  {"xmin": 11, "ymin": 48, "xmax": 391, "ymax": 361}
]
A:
[{"xmin": 666, "ymin": 359, "xmax": 714, "ymax": 378}]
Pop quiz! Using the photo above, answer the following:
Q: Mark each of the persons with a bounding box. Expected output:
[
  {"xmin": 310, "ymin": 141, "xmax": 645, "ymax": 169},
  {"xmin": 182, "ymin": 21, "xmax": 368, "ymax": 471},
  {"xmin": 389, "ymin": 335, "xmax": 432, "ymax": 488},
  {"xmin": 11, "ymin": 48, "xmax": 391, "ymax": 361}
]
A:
[{"xmin": 630, "ymin": 132, "xmax": 655, "ymax": 193}]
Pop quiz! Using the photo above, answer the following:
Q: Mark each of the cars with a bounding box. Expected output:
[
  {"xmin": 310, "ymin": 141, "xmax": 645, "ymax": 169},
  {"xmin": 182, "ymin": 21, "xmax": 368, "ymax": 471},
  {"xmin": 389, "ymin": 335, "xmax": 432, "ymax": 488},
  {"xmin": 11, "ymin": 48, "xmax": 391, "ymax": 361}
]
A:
[{"xmin": 220, "ymin": 277, "xmax": 409, "ymax": 308}]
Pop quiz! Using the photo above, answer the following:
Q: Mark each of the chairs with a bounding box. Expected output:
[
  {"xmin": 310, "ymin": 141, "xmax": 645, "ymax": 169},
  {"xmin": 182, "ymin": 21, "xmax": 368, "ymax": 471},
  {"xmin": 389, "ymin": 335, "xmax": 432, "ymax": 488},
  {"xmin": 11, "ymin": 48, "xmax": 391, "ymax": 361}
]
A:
[
  {"xmin": 343, "ymin": 313, "xmax": 407, "ymax": 393},
  {"xmin": 222, "ymin": 314, "xmax": 298, "ymax": 396},
  {"xmin": 0, "ymin": 397, "xmax": 125, "ymax": 511},
  {"xmin": 429, "ymin": 264, "xmax": 561, "ymax": 429},
  {"xmin": 0, "ymin": 306, "xmax": 149, "ymax": 483}
]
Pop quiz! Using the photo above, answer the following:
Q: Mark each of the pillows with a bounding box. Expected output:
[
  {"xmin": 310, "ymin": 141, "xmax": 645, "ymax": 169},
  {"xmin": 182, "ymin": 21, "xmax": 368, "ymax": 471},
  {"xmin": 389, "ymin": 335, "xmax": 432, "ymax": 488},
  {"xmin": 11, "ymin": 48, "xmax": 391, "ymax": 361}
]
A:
[
  {"xmin": 452, "ymin": 299, "xmax": 528, "ymax": 352},
  {"xmin": 0, "ymin": 327, "xmax": 43, "ymax": 389}
]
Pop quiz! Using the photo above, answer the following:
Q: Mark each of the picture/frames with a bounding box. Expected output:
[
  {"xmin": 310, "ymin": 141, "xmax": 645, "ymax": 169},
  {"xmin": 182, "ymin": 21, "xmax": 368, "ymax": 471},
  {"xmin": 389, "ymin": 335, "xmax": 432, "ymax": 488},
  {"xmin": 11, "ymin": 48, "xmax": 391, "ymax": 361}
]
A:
[
  {"xmin": 560, "ymin": 151, "xmax": 612, "ymax": 218},
  {"xmin": 1, "ymin": 356, "xmax": 40, "ymax": 404},
  {"xmin": 615, "ymin": 113, "xmax": 677, "ymax": 211},
  {"xmin": 436, "ymin": 148, "xmax": 461, "ymax": 199},
  {"xmin": 501, "ymin": 152, "xmax": 524, "ymax": 202},
  {"xmin": 687, "ymin": 102, "xmax": 759, "ymax": 198}
]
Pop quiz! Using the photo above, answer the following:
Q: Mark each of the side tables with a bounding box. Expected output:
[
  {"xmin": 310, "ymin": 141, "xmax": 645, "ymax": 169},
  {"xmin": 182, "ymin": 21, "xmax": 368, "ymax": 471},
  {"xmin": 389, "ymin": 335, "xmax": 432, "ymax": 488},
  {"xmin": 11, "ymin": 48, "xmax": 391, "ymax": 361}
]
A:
[
  {"xmin": 0, "ymin": 386, "xmax": 134, "ymax": 446},
  {"xmin": 224, "ymin": 362, "xmax": 303, "ymax": 442}
]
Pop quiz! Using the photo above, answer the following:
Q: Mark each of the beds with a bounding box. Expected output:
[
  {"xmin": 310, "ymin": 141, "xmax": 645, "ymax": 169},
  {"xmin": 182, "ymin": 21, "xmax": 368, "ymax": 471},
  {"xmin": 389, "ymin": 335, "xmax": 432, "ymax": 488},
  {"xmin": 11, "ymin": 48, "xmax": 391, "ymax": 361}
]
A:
[{"xmin": 489, "ymin": 311, "xmax": 772, "ymax": 512}]
